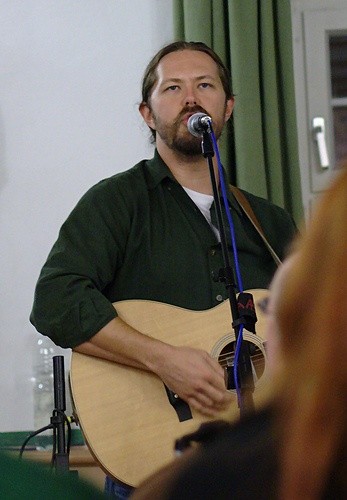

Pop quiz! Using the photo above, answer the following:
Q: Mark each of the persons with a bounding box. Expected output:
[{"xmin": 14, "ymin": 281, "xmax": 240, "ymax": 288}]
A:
[{"xmin": 28, "ymin": 38, "xmax": 300, "ymax": 500}]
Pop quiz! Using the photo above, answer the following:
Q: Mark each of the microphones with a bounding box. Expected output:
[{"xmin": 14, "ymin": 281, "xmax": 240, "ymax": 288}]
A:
[{"xmin": 187, "ymin": 112, "xmax": 212, "ymax": 138}]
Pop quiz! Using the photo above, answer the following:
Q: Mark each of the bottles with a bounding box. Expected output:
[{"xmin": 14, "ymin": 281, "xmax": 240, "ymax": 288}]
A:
[{"xmin": 30, "ymin": 334, "xmax": 69, "ymax": 454}]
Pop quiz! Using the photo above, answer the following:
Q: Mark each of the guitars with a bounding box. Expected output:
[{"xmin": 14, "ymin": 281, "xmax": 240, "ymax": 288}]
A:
[{"xmin": 71, "ymin": 288, "xmax": 277, "ymax": 491}]
